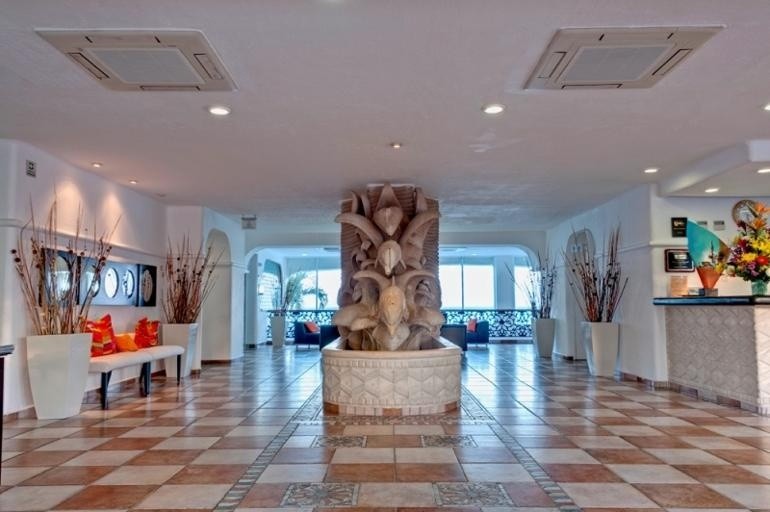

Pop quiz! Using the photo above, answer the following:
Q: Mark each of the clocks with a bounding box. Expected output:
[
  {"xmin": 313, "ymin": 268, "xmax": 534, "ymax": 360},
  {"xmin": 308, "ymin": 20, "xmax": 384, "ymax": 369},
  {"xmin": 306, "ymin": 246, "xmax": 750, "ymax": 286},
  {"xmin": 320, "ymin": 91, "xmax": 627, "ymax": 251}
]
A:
[{"xmin": 733, "ymin": 198, "xmax": 758, "ymax": 227}]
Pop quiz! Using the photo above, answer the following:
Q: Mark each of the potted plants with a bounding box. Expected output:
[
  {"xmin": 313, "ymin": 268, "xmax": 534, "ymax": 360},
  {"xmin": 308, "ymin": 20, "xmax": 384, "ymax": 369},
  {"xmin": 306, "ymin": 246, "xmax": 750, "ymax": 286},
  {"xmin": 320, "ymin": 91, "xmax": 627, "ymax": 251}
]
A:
[{"xmin": 268, "ymin": 267, "xmax": 329, "ymax": 350}]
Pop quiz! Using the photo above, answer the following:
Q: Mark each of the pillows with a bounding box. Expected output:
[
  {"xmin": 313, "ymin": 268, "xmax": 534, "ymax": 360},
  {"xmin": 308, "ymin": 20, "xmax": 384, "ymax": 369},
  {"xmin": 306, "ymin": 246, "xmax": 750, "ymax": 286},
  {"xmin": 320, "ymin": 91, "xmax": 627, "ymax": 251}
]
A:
[
  {"xmin": 467, "ymin": 319, "xmax": 477, "ymax": 332},
  {"xmin": 115, "ymin": 333, "xmax": 141, "ymax": 353},
  {"xmin": 305, "ymin": 321, "xmax": 322, "ymax": 333},
  {"xmin": 134, "ymin": 315, "xmax": 162, "ymax": 348},
  {"xmin": 83, "ymin": 310, "xmax": 118, "ymax": 359}
]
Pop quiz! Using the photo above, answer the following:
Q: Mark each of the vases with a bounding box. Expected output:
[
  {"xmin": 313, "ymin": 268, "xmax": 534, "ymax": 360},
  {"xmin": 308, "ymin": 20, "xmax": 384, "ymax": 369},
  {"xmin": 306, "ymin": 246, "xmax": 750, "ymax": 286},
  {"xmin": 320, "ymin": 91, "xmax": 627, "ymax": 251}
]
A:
[
  {"xmin": 750, "ymin": 279, "xmax": 769, "ymax": 296},
  {"xmin": 161, "ymin": 321, "xmax": 200, "ymax": 378},
  {"xmin": 697, "ymin": 266, "xmax": 722, "ymax": 291},
  {"xmin": 580, "ymin": 320, "xmax": 621, "ymax": 378},
  {"xmin": 531, "ymin": 316, "xmax": 557, "ymax": 359},
  {"xmin": 25, "ymin": 331, "xmax": 93, "ymax": 421}
]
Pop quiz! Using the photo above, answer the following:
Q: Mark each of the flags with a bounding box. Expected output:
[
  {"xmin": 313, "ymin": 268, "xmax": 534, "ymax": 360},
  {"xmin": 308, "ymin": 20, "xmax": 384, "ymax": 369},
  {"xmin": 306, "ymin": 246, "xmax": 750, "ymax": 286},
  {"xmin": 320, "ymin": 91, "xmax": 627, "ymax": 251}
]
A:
[{"xmin": 687, "ymin": 220, "xmax": 730, "ymax": 289}]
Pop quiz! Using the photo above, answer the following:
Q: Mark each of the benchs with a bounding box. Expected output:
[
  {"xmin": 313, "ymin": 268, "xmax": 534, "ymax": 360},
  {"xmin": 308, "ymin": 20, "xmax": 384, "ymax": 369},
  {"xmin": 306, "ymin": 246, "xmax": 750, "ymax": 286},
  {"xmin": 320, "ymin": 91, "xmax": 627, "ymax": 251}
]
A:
[{"xmin": 87, "ymin": 344, "xmax": 186, "ymax": 410}]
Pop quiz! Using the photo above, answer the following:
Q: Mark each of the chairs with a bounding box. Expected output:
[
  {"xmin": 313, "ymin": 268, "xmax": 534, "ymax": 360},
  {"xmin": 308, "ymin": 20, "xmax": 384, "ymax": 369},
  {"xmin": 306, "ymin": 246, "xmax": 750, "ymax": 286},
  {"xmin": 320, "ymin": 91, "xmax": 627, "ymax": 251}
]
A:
[
  {"xmin": 440, "ymin": 322, "xmax": 468, "ymax": 355},
  {"xmin": 467, "ymin": 320, "xmax": 491, "ymax": 349},
  {"xmin": 293, "ymin": 320, "xmax": 322, "ymax": 350},
  {"xmin": 320, "ymin": 324, "xmax": 340, "ymax": 351}
]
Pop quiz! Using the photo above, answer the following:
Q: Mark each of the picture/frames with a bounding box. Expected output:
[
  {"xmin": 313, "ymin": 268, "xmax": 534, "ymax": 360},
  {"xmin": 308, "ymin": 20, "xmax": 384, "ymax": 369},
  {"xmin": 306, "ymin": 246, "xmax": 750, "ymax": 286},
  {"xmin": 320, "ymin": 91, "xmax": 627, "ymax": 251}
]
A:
[{"xmin": 664, "ymin": 248, "xmax": 695, "ymax": 273}]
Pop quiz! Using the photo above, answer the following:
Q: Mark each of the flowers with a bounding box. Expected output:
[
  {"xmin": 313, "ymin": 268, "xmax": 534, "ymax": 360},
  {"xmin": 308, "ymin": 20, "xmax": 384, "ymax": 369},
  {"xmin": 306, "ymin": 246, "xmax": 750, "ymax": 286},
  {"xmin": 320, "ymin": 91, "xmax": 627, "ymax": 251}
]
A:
[
  {"xmin": 697, "ymin": 244, "xmax": 734, "ymax": 278},
  {"xmin": 710, "ymin": 198, "xmax": 770, "ymax": 285}
]
